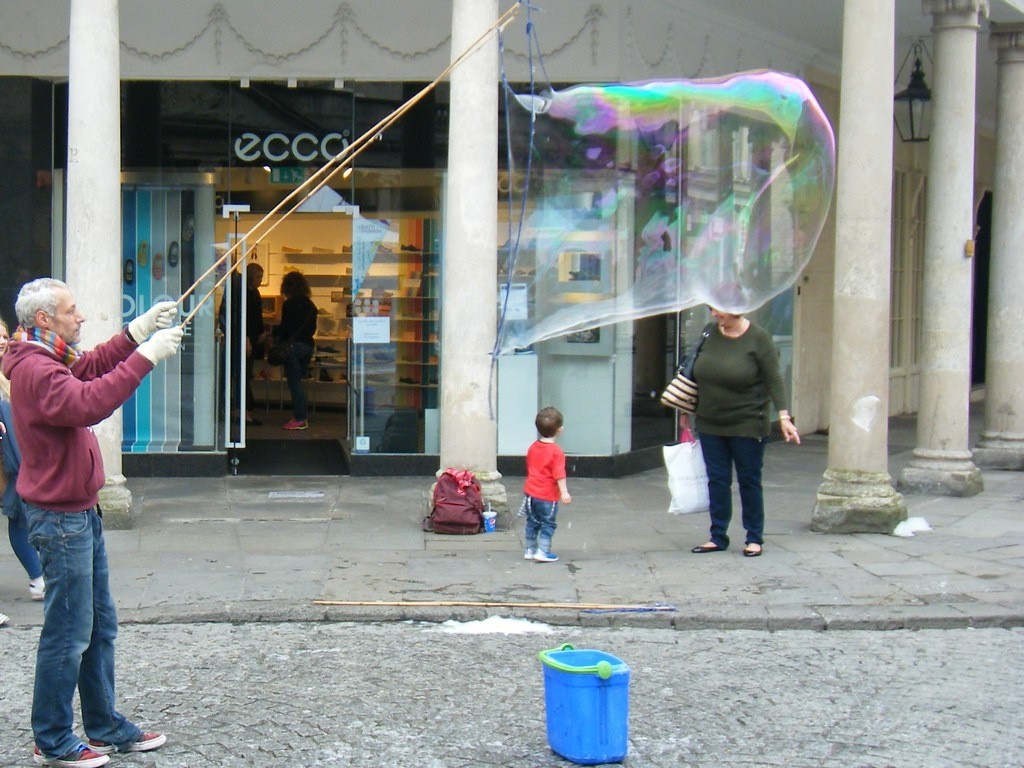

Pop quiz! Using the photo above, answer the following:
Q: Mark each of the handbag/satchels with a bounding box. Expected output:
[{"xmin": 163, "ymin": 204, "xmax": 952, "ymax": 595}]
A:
[
  {"xmin": 268, "ymin": 340, "xmax": 295, "ymax": 365},
  {"xmin": 660, "ymin": 323, "xmax": 716, "ymax": 415},
  {"xmin": 663, "ymin": 429, "xmax": 710, "ymax": 514}
]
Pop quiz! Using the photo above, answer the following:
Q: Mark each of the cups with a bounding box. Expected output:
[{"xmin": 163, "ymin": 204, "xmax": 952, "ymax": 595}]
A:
[{"xmin": 483, "ymin": 511, "xmax": 497, "ymax": 533}]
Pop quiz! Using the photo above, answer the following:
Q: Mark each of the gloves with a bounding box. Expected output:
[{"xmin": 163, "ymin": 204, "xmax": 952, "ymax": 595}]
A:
[
  {"xmin": 128, "ymin": 301, "xmax": 179, "ymax": 346},
  {"xmin": 136, "ymin": 326, "xmax": 183, "ymax": 367}
]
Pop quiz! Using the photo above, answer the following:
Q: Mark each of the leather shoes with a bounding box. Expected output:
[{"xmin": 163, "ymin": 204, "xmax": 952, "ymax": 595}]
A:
[
  {"xmin": 744, "ymin": 542, "xmax": 761, "ymax": 556},
  {"xmin": 692, "ymin": 542, "xmax": 720, "ymax": 553}
]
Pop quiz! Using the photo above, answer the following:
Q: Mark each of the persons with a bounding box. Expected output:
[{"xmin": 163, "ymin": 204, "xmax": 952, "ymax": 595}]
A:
[
  {"xmin": 523, "ymin": 406, "xmax": 572, "ymax": 562},
  {"xmin": 1, "ymin": 278, "xmax": 186, "ymax": 768},
  {"xmin": 679, "ymin": 284, "xmax": 801, "ymax": 555},
  {"xmin": 231, "ymin": 262, "xmax": 265, "ymax": 426},
  {"xmin": 0, "ymin": 318, "xmax": 46, "ymax": 623},
  {"xmin": 272, "ymin": 271, "xmax": 319, "ymax": 431}
]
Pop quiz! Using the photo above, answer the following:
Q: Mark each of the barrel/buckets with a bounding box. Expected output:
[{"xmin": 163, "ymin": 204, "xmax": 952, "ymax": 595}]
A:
[{"xmin": 538, "ymin": 642, "xmax": 633, "ymax": 765}]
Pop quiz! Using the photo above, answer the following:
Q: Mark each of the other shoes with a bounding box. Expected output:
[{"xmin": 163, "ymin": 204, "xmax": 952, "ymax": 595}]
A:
[
  {"xmin": 236, "ymin": 417, "xmax": 262, "ymax": 426},
  {"xmin": 29, "ymin": 581, "xmax": 46, "ymax": 599},
  {"xmin": 253, "ymin": 244, "xmax": 438, "ymax": 385}
]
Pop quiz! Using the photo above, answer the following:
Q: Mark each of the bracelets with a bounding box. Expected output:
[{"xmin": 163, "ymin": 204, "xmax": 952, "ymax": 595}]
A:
[{"xmin": 779, "ymin": 415, "xmax": 791, "ymax": 420}]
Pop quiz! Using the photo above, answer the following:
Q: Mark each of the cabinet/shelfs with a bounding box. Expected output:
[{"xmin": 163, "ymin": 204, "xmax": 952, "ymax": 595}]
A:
[
  {"xmin": 501, "ymin": 236, "xmax": 613, "ymax": 354},
  {"xmin": 253, "ymin": 249, "xmax": 440, "ymax": 411}
]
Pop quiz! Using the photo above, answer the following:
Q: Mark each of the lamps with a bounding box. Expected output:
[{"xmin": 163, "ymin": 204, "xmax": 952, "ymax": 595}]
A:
[
  {"xmin": 893, "ymin": 41, "xmax": 933, "ymax": 141},
  {"xmin": 244, "ymin": 167, "xmax": 256, "ymax": 185},
  {"xmin": 339, "ymin": 166, "xmax": 353, "ymax": 179}
]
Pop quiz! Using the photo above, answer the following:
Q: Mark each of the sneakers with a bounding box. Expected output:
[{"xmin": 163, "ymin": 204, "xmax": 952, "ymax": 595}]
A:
[
  {"xmin": 534, "ymin": 548, "xmax": 558, "ymax": 561},
  {"xmin": 524, "ymin": 546, "xmax": 539, "ymax": 559},
  {"xmin": 281, "ymin": 417, "xmax": 308, "ymax": 429},
  {"xmin": 33, "ymin": 745, "xmax": 110, "ymax": 768},
  {"xmin": 89, "ymin": 732, "xmax": 166, "ymax": 754}
]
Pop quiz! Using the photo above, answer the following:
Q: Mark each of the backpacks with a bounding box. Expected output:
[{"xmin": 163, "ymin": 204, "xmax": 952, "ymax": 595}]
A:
[{"xmin": 422, "ymin": 468, "xmax": 486, "ymax": 535}]
